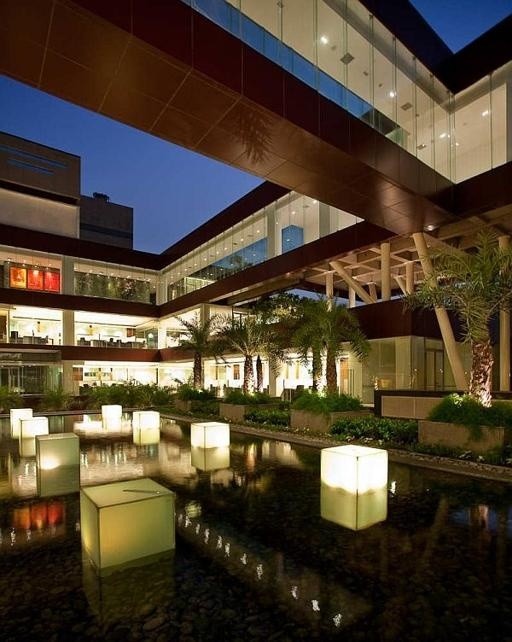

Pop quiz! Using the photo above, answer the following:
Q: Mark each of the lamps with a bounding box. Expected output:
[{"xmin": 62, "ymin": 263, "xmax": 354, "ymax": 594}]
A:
[
  {"xmin": 102, "ymin": 405, "xmax": 122, "ymax": 417},
  {"xmin": 320, "ymin": 443, "xmax": 388, "ymax": 493},
  {"xmin": 37, "ymin": 433, "xmax": 80, "ymax": 468},
  {"xmin": 132, "ymin": 410, "xmax": 160, "ymax": 430},
  {"xmin": 9, "ymin": 408, "xmax": 32, "ymax": 439},
  {"xmin": 191, "ymin": 421, "xmax": 230, "ymax": 448},
  {"xmin": 80, "ymin": 478, "xmax": 176, "ymax": 579},
  {"xmin": 18, "ymin": 417, "xmax": 49, "ymax": 439}
]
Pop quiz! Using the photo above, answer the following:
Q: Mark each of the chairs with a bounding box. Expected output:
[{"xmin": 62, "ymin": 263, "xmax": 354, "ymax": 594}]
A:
[{"xmin": 12, "ymin": 330, "xmax": 146, "ymax": 349}]
